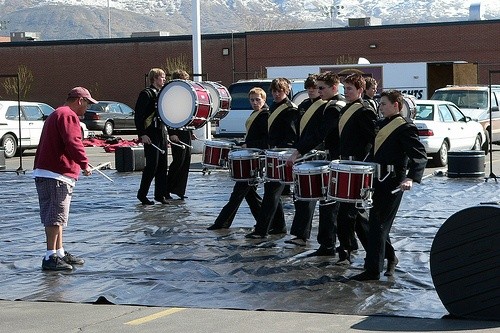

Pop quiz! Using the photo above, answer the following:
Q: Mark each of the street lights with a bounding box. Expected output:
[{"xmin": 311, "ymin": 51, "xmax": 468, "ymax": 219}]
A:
[{"xmin": 320, "ymin": 5, "xmax": 344, "ymax": 27}]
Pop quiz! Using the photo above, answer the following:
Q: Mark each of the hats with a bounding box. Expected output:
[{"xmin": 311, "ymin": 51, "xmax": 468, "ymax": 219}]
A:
[{"xmin": 68, "ymin": 86, "xmax": 99, "ymax": 104}]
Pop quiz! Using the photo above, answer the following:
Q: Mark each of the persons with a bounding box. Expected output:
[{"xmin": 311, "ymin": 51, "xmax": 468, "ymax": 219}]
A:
[
  {"xmin": 167, "ymin": 69, "xmax": 198, "ymax": 199},
  {"xmin": 206, "ymin": 70, "xmax": 427, "ymax": 280},
  {"xmin": 32, "ymin": 87, "xmax": 92, "ymax": 271},
  {"xmin": 133, "ymin": 68, "xmax": 170, "ymax": 205}
]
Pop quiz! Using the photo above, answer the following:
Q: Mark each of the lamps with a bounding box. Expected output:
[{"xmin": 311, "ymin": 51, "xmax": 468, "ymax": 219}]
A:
[{"xmin": 369, "ymin": 44, "xmax": 377, "ymax": 49}]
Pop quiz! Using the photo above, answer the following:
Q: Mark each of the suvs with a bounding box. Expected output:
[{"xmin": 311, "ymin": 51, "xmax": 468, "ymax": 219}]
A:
[
  {"xmin": 419, "ymin": 84, "xmax": 500, "ymax": 144},
  {"xmin": 215, "ymin": 78, "xmax": 346, "ymax": 141}
]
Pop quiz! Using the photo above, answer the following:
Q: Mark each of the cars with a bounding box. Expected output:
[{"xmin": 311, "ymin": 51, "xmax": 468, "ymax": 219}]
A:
[
  {"xmin": 78, "ymin": 101, "xmax": 137, "ymax": 137},
  {"xmin": 0, "ymin": 100, "xmax": 88, "ymax": 158},
  {"xmin": 413, "ymin": 99, "xmax": 486, "ymax": 167}
]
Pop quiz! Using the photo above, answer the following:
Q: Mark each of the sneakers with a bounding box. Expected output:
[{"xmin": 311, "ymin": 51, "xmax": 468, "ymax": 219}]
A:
[{"xmin": 41, "ymin": 250, "xmax": 84, "ymax": 270}]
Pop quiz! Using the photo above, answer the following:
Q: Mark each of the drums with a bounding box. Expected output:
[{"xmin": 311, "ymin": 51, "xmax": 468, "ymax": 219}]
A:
[
  {"xmin": 264, "ymin": 147, "xmax": 297, "ymax": 183},
  {"xmin": 201, "ymin": 140, "xmax": 236, "ymax": 169},
  {"xmin": 327, "ymin": 160, "xmax": 375, "ymax": 203},
  {"xmin": 200, "ymin": 81, "xmax": 232, "ymax": 122},
  {"xmin": 292, "ymin": 160, "xmax": 331, "ymax": 202},
  {"xmin": 228, "ymin": 148, "xmax": 260, "ymax": 182},
  {"xmin": 155, "ymin": 79, "xmax": 214, "ymax": 130}
]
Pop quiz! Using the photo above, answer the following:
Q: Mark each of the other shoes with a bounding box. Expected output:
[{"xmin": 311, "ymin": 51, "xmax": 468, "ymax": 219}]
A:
[
  {"xmin": 284, "ymin": 237, "xmax": 306, "ymax": 246},
  {"xmin": 245, "ymin": 232, "xmax": 268, "ymax": 238},
  {"xmin": 269, "ymin": 228, "xmax": 287, "ymax": 234},
  {"xmin": 154, "ymin": 195, "xmax": 169, "ymax": 203},
  {"xmin": 335, "ymin": 244, "xmax": 359, "ymax": 252},
  {"xmin": 349, "ymin": 271, "xmax": 380, "ymax": 281},
  {"xmin": 175, "ymin": 193, "xmax": 189, "ymax": 198},
  {"xmin": 207, "ymin": 224, "xmax": 229, "ymax": 230},
  {"xmin": 307, "ymin": 246, "xmax": 336, "ymax": 256},
  {"xmin": 164, "ymin": 193, "xmax": 173, "ymax": 199},
  {"xmin": 136, "ymin": 194, "xmax": 154, "ymax": 205},
  {"xmin": 384, "ymin": 256, "xmax": 399, "ymax": 276}
]
